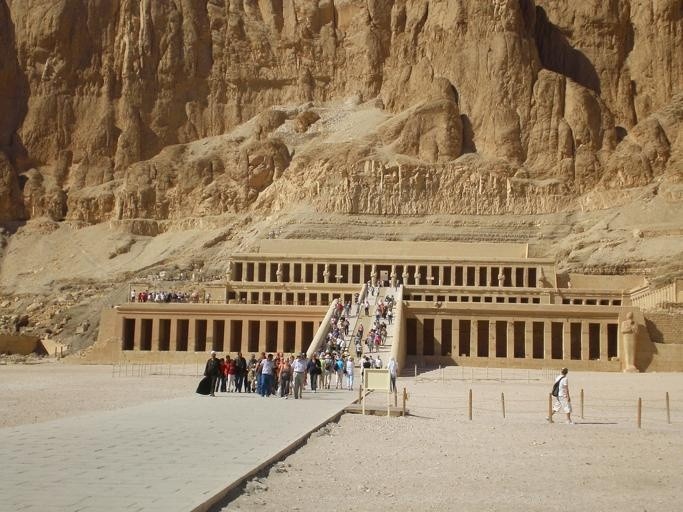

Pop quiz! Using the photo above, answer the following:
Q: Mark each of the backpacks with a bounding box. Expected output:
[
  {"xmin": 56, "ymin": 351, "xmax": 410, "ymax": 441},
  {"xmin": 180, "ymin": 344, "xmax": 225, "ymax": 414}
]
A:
[{"xmin": 552, "ymin": 382, "xmax": 559, "ymax": 396}]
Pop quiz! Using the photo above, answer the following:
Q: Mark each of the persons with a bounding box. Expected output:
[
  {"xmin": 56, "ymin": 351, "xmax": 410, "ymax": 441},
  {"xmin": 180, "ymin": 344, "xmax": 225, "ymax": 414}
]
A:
[
  {"xmin": 204, "ymin": 279, "xmax": 398, "ymax": 400},
  {"xmin": 132, "ymin": 288, "xmax": 211, "ymax": 303},
  {"xmin": 545, "ymin": 368, "xmax": 574, "ymax": 425},
  {"xmin": 620, "ymin": 312, "xmax": 639, "ymax": 370}
]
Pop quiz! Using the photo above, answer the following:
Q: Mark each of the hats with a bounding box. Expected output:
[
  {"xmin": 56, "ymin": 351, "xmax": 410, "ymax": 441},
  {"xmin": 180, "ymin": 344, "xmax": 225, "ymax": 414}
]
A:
[{"xmin": 562, "ymin": 367, "xmax": 568, "ymax": 370}]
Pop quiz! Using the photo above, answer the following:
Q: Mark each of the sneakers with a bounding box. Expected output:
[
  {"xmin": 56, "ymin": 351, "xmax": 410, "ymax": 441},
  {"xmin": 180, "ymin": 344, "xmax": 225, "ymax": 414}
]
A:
[
  {"xmin": 546, "ymin": 416, "xmax": 553, "ymax": 423},
  {"xmin": 566, "ymin": 421, "xmax": 574, "ymax": 424}
]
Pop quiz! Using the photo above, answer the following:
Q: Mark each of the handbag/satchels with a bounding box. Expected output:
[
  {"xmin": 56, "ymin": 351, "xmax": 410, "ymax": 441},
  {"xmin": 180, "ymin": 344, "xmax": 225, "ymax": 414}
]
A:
[{"xmin": 196, "ymin": 377, "xmax": 210, "ymax": 395}]
